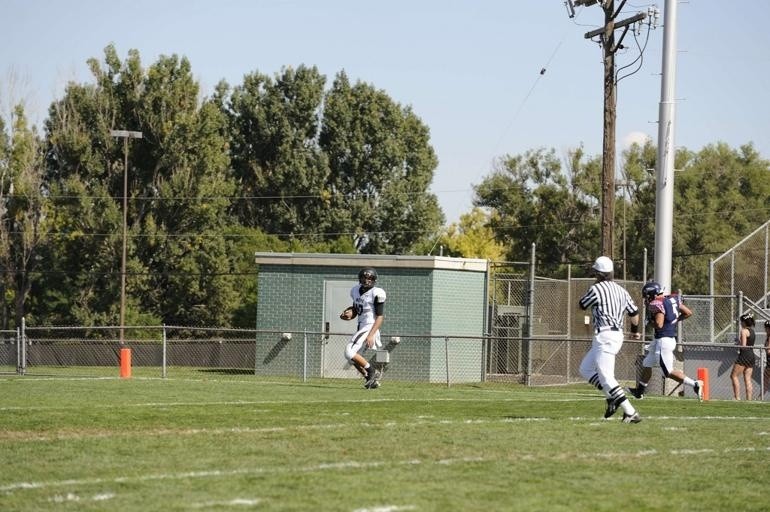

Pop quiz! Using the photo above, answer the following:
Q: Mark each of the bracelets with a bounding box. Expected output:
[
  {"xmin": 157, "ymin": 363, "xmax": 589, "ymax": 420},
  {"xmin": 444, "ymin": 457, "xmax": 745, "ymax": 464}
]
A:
[{"xmin": 630, "ymin": 322, "xmax": 640, "ymax": 334}]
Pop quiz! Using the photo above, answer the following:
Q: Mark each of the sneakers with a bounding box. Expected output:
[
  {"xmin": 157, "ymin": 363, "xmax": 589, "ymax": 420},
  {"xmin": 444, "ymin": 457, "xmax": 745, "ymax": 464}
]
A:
[
  {"xmin": 625, "ymin": 388, "xmax": 643, "ymax": 400},
  {"xmin": 693, "ymin": 381, "xmax": 704, "ymax": 402},
  {"xmin": 604, "ymin": 398, "xmax": 619, "ymax": 418},
  {"xmin": 621, "ymin": 412, "xmax": 642, "ymax": 424},
  {"xmin": 364, "ymin": 364, "xmax": 381, "ymax": 389}
]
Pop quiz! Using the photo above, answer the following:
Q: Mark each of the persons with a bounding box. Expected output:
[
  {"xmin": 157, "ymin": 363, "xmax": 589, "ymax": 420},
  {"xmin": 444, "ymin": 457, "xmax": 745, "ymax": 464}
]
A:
[
  {"xmin": 730, "ymin": 314, "xmax": 756, "ymax": 402},
  {"xmin": 624, "ymin": 281, "xmax": 704, "ymax": 401},
  {"xmin": 340, "ymin": 269, "xmax": 388, "ymax": 388},
  {"xmin": 578, "ymin": 257, "xmax": 642, "ymax": 423},
  {"xmin": 757, "ymin": 320, "xmax": 770, "ymax": 401}
]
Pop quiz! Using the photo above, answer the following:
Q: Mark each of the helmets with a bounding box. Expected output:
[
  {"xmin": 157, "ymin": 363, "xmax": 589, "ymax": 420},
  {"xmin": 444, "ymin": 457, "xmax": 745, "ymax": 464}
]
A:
[
  {"xmin": 358, "ymin": 268, "xmax": 378, "ymax": 287},
  {"xmin": 642, "ymin": 283, "xmax": 661, "ymax": 298}
]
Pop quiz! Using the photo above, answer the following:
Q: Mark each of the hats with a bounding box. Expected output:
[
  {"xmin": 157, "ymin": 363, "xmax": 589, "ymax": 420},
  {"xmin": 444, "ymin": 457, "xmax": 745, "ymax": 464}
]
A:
[{"xmin": 592, "ymin": 256, "xmax": 614, "ymax": 272}]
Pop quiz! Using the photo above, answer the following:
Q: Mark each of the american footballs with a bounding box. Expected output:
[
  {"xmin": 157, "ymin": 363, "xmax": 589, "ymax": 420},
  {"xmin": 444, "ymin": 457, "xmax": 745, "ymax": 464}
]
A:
[{"xmin": 344, "ymin": 307, "xmax": 356, "ymax": 319}]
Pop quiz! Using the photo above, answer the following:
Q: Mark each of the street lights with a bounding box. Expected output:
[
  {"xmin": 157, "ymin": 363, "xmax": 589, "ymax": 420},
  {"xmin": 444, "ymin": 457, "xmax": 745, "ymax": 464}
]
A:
[{"xmin": 111, "ymin": 130, "xmax": 145, "ymax": 343}]
